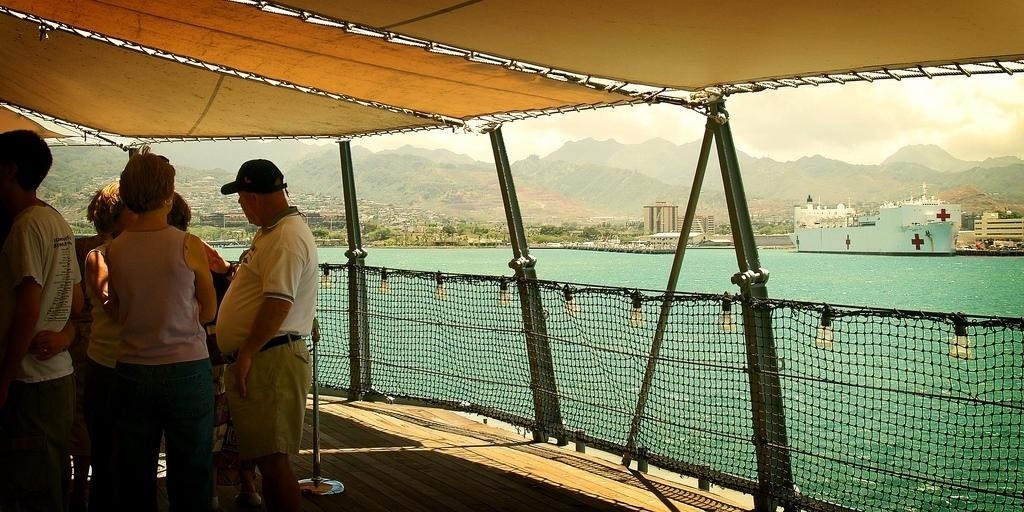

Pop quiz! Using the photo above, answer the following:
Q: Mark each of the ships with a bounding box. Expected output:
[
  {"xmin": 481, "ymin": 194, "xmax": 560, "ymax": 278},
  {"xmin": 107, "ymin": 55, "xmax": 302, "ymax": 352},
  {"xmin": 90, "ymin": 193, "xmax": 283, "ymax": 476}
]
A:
[{"xmin": 788, "ymin": 181, "xmax": 961, "ymax": 256}]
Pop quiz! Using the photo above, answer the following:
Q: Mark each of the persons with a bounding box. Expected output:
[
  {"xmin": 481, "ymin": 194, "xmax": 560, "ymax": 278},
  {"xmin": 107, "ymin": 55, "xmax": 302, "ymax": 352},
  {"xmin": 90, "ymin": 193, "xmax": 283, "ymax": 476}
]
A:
[
  {"xmin": 214, "ymin": 159, "xmax": 320, "ymax": 511},
  {"xmin": 83, "ymin": 153, "xmax": 228, "ymax": 512},
  {"xmin": 1, "ymin": 130, "xmax": 85, "ymax": 512},
  {"xmin": 966, "ymin": 237, "xmax": 994, "ymax": 250}
]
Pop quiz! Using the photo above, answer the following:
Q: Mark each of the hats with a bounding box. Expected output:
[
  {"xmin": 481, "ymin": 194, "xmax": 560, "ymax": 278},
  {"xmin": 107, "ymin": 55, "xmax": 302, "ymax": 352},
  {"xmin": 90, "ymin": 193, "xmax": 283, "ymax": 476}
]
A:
[{"xmin": 221, "ymin": 159, "xmax": 287, "ymax": 195}]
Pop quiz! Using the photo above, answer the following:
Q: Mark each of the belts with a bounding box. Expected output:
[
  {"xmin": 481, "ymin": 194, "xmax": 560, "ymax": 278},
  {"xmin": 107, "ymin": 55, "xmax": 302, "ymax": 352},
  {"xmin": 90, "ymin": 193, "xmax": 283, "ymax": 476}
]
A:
[{"xmin": 258, "ymin": 334, "xmax": 301, "ymax": 353}]
